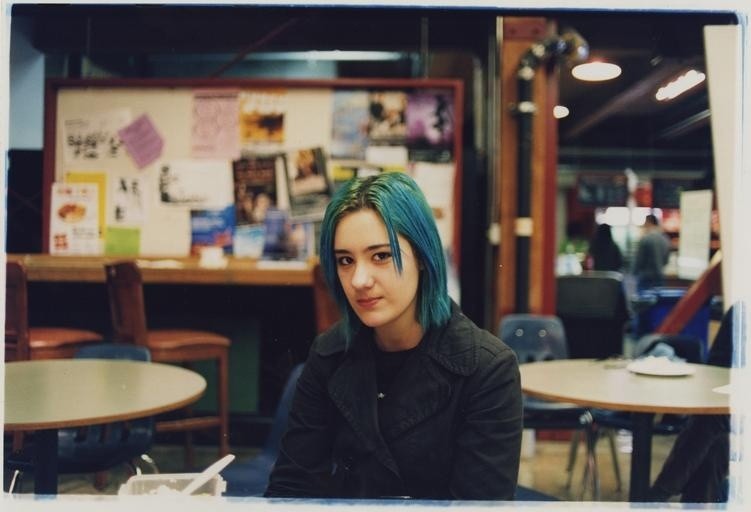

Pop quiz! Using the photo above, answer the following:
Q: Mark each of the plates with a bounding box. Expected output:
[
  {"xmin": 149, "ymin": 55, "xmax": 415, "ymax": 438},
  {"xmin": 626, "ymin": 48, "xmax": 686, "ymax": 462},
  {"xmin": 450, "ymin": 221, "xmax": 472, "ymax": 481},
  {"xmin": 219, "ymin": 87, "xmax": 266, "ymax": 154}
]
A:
[{"xmin": 626, "ymin": 356, "xmax": 695, "ymax": 377}]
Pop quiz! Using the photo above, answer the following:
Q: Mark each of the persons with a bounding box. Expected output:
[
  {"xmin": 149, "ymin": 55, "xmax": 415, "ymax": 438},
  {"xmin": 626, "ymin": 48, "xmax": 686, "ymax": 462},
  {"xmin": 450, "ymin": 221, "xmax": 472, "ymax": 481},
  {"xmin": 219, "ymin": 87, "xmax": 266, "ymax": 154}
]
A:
[
  {"xmin": 630, "ymin": 214, "xmax": 672, "ymax": 320},
  {"xmin": 641, "ymin": 302, "xmax": 749, "ymax": 500},
  {"xmin": 289, "ymin": 148, "xmax": 334, "ymax": 218},
  {"xmin": 370, "ymin": 103, "xmax": 405, "ymax": 140},
  {"xmin": 261, "ymin": 216, "xmax": 293, "ymax": 261},
  {"xmin": 585, "ymin": 223, "xmax": 624, "ymax": 273},
  {"xmin": 287, "ymin": 222, "xmax": 314, "ymax": 262},
  {"xmin": 159, "ymin": 163, "xmax": 198, "ymax": 204},
  {"xmin": 261, "ymin": 171, "xmax": 527, "ymax": 502},
  {"xmin": 430, "ymin": 94, "xmax": 448, "ymax": 143}
]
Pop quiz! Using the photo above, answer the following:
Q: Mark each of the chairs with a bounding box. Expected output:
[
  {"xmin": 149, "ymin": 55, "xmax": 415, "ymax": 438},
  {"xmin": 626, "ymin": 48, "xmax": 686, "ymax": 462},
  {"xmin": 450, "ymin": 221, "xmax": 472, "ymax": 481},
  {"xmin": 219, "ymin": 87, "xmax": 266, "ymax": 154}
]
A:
[
  {"xmin": 498, "ymin": 273, "xmax": 626, "ymax": 499},
  {"xmin": 3, "ymin": 258, "xmax": 232, "ymax": 493}
]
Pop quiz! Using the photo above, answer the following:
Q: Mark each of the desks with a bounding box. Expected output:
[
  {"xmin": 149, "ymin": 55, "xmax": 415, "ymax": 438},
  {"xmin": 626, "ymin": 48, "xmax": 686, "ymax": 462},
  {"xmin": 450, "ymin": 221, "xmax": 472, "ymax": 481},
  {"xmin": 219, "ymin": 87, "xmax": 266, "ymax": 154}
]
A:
[{"xmin": 515, "ymin": 356, "xmax": 732, "ymax": 504}]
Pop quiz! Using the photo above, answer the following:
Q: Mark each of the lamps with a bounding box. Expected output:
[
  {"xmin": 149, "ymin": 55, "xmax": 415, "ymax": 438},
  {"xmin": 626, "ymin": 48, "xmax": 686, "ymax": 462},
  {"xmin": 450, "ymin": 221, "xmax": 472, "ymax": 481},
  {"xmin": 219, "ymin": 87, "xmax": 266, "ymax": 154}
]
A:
[{"xmin": 569, "ymin": 40, "xmax": 623, "ymax": 82}]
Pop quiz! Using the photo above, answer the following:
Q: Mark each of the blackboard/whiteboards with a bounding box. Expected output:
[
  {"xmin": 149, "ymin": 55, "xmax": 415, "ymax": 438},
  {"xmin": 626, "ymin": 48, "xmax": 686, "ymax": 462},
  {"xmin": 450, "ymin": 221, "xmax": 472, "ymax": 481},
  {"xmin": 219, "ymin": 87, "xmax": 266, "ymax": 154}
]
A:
[{"xmin": 43, "ymin": 77, "xmax": 465, "ymax": 267}]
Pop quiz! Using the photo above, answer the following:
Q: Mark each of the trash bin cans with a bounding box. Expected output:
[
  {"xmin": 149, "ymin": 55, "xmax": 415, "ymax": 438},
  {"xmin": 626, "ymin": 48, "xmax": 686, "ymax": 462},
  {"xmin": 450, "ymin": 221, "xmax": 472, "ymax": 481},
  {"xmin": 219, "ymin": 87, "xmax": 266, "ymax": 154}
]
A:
[{"xmin": 555, "ymin": 273, "xmax": 716, "ymax": 366}]
